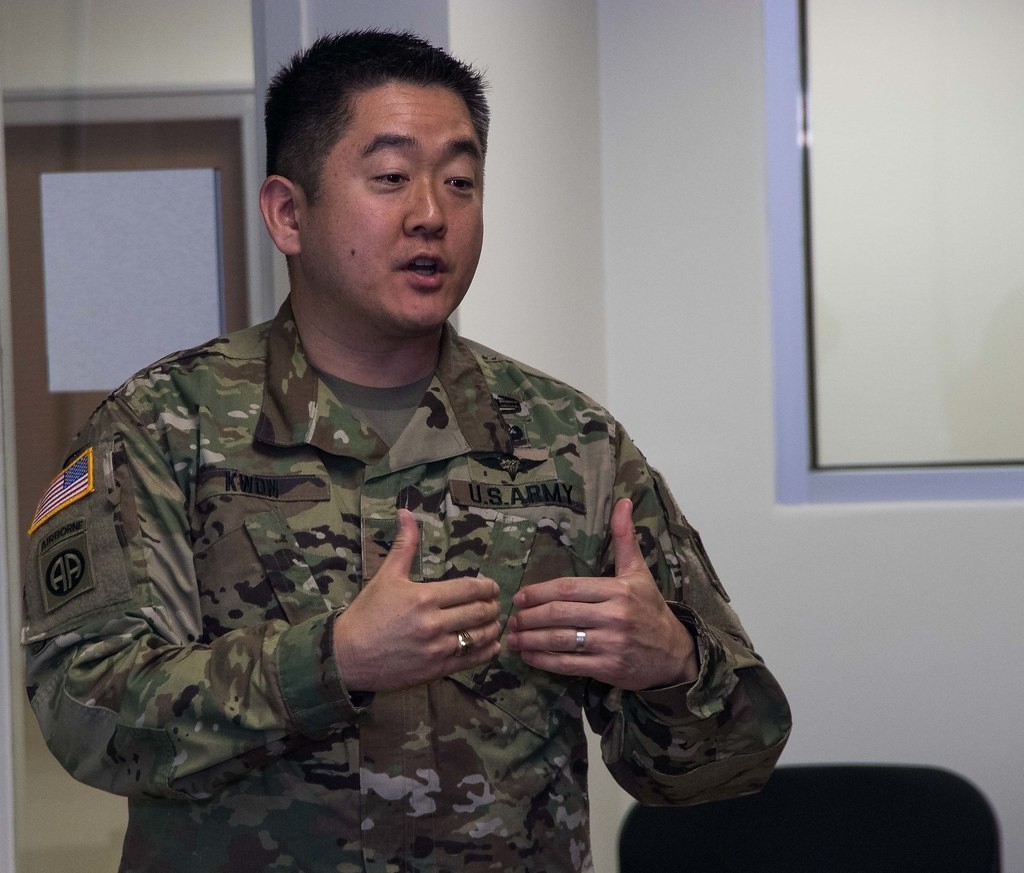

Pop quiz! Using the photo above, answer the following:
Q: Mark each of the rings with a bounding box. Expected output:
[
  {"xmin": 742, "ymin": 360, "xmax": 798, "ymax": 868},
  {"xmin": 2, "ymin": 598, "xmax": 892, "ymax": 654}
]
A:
[
  {"xmin": 454, "ymin": 630, "xmax": 477, "ymax": 659},
  {"xmin": 574, "ymin": 630, "xmax": 588, "ymax": 651}
]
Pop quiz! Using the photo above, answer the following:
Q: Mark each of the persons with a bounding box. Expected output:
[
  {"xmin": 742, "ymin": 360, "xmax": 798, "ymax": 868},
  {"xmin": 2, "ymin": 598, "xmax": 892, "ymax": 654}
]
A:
[{"xmin": 16, "ymin": 29, "xmax": 792, "ymax": 873}]
